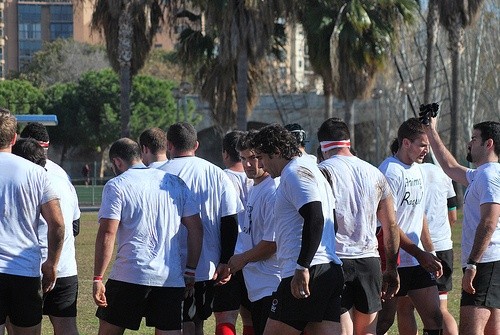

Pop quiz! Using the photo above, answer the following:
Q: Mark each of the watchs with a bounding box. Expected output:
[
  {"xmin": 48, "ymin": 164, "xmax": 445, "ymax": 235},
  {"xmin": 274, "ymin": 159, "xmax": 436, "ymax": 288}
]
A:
[{"xmin": 466, "ymin": 258, "xmax": 478, "ymax": 265}]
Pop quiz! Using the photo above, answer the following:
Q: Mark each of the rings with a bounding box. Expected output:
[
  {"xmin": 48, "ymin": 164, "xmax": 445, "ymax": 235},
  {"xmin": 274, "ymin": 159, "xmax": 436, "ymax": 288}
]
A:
[{"xmin": 299, "ymin": 289, "xmax": 306, "ymax": 296}]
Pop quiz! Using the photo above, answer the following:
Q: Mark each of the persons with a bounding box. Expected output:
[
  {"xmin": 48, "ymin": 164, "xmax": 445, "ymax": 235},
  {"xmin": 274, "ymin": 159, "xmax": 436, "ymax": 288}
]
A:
[
  {"xmin": 5, "ymin": 138, "xmax": 81, "ymax": 335},
  {"xmin": 91, "ymin": 116, "xmax": 460, "ymax": 335},
  {"xmin": 0, "ymin": 108, "xmax": 65, "ymax": 335},
  {"xmin": 21, "ymin": 121, "xmax": 70, "ymax": 180},
  {"xmin": 83, "ymin": 161, "xmax": 90, "ymax": 186},
  {"xmin": 420, "ymin": 114, "xmax": 500, "ymax": 335}
]
